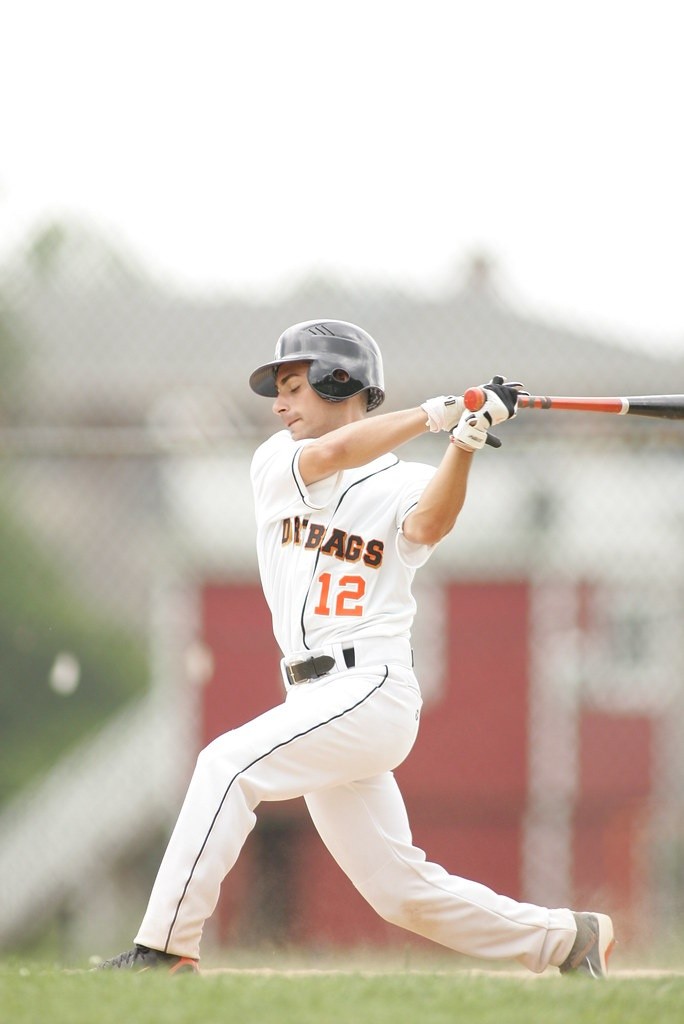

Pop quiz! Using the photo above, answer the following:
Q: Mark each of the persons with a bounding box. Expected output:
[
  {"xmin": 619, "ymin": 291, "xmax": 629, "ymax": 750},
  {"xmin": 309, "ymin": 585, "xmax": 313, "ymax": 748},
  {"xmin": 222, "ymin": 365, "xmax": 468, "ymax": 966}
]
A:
[{"xmin": 100, "ymin": 319, "xmax": 614, "ymax": 978}]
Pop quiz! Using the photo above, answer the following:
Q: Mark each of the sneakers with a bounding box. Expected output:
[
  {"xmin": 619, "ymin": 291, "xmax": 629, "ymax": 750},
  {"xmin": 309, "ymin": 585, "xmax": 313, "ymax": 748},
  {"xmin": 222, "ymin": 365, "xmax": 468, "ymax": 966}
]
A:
[
  {"xmin": 559, "ymin": 912, "xmax": 615, "ymax": 979},
  {"xmin": 87, "ymin": 944, "xmax": 200, "ymax": 975}
]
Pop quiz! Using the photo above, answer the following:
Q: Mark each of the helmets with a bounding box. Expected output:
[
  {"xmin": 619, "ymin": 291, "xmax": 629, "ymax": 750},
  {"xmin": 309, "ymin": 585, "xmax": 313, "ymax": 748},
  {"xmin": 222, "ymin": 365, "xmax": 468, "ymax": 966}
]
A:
[{"xmin": 249, "ymin": 318, "xmax": 386, "ymax": 411}]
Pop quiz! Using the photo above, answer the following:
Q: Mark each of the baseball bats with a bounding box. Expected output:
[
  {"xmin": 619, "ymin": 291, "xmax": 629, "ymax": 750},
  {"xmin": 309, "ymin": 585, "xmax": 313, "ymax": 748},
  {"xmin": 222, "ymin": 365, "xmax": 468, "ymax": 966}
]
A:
[{"xmin": 463, "ymin": 385, "xmax": 684, "ymax": 421}]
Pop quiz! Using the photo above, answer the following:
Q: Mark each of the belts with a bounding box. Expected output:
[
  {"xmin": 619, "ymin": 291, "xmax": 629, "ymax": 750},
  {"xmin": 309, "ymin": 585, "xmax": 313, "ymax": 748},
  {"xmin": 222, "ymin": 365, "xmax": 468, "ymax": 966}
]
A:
[{"xmin": 285, "ymin": 648, "xmax": 414, "ymax": 683}]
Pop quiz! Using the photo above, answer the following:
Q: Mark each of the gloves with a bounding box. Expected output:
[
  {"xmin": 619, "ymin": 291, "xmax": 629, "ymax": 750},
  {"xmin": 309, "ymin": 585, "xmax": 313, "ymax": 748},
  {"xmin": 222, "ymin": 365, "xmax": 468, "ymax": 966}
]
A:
[{"xmin": 421, "ymin": 374, "xmax": 529, "ymax": 449}]
